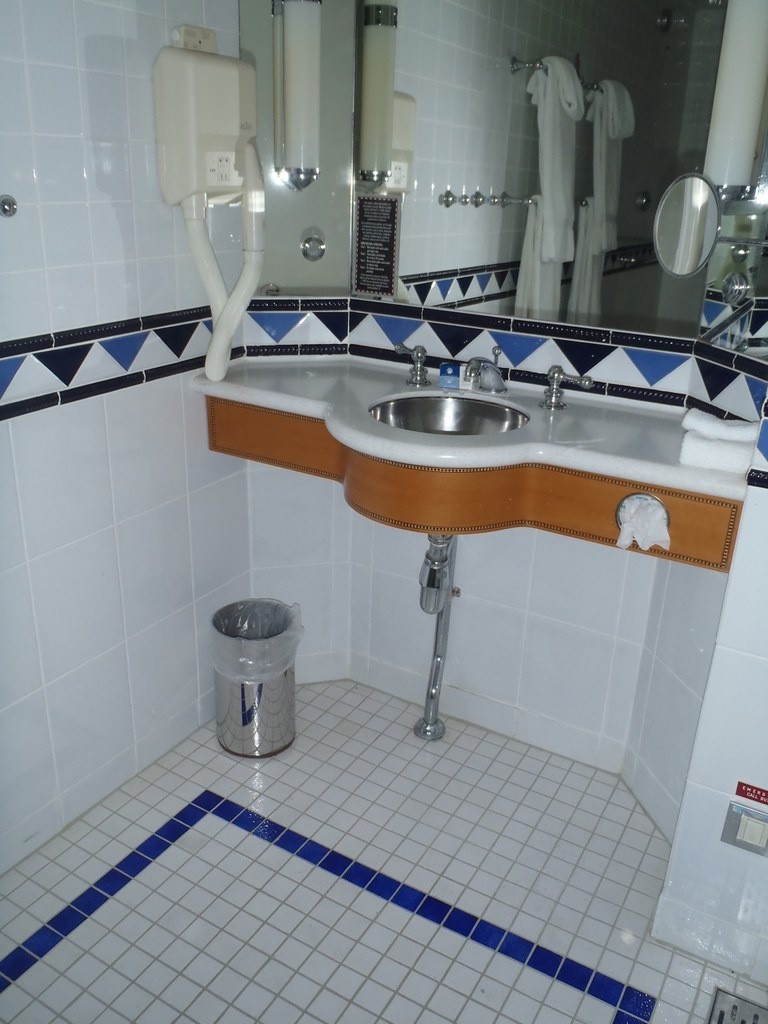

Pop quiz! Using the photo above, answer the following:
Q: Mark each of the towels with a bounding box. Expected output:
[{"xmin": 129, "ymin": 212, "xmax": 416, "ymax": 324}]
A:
[
  {"xmin": 677, "ymin": 407, "xmax": 762, "ymax": 474},
  {"xmin": 512, "ymin": 193, "xmax": 604, "ymax": 327},
  {"xmin": 525, "ymin": 56, "xmax": 636, "ymax": 266}
]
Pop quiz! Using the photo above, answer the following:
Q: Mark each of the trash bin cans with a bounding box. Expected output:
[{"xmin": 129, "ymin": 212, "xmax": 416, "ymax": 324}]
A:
[{"xmin": 212, "ymin": 598, "xmax": 296, "ymax": 758}]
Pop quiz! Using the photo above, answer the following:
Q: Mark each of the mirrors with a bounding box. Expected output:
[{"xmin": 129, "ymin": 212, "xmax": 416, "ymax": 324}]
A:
[
  {"xmin": 654, "ymin": 173, "xmax": 768, "ymax": 279},
  {"xmin": 238, "ymin": 0, "xmax": 768, "ymax": 362}
]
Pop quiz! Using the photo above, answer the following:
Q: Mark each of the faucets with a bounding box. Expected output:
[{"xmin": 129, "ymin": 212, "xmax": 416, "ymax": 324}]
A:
[{"xmin": 464, "ymin": 356, "xmax": 508, "ymax": 397}]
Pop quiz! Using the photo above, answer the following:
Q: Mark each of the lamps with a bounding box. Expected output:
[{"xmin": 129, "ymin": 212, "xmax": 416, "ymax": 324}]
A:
[
  {"xmin": 354, "ymin": 0, "xmax": 398, "ymax": 191},
  {"xmin": 272, "ymin": 0, "xmax": 322, "ymax": 192}
]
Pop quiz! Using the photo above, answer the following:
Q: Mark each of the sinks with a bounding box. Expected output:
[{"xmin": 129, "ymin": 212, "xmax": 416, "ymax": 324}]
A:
[{"xmin": 366, "ymin": 377, "xmax": 532, "ymax": 437}]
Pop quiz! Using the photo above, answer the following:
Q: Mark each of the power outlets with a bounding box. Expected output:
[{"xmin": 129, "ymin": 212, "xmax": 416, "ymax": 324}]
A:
[
  {"xmin": 386, "ymin": 161, "xmax": 408, "ymax": 188},
  {"xmin": 207, "ymin": 151, "xmax": 243, "ymax": 186}
]
